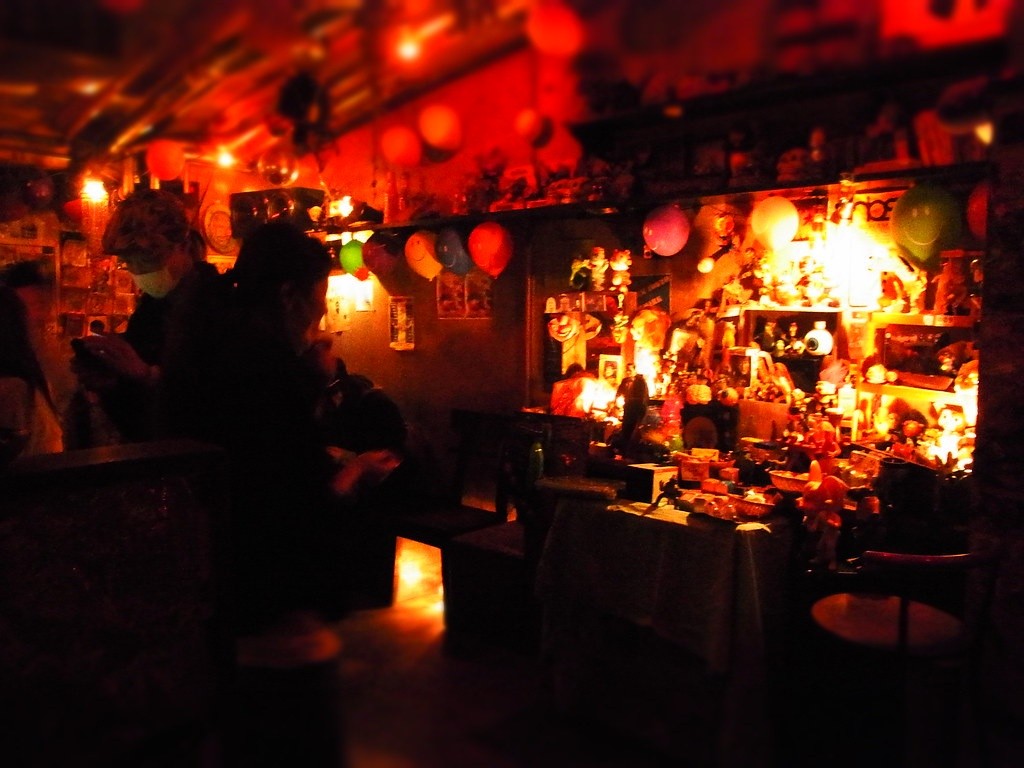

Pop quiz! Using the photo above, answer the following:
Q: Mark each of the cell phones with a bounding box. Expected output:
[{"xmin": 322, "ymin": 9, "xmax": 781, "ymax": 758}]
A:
[{"xmin": 71, "ymin": 340, "xmax": 99, "ymax": 367}]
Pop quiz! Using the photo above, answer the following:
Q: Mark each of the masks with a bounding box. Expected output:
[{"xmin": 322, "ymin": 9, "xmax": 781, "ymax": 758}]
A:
[{"xmin": 128, "ymin": 248, "xmax": 190, "ymax": 300}]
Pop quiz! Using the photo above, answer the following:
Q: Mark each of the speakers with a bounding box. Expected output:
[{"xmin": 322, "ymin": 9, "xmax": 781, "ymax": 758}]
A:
[{"xmin": 229, "ymin": 187, "xmax": 331, "ymax": 240}]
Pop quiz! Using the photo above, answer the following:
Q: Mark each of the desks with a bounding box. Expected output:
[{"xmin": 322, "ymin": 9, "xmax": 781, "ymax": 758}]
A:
[{"xmin": 546, "ymin": 498, "xmax": 789, "ymax": 767}]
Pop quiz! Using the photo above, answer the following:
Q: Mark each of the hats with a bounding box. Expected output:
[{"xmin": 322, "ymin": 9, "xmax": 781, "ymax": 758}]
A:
[{"xmin": 101, "ymin": 189, "xmax": 190, "ymax": 254}]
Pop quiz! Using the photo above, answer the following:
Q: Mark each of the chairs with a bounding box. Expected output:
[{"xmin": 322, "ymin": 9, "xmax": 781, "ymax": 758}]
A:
[
  {"xmin": 401, "ymin": 412, "xmax": 582, "ymax": 646},
  {"xmin": 806, "ymin": 526, "xmax": 1005, "ymax": 768}
]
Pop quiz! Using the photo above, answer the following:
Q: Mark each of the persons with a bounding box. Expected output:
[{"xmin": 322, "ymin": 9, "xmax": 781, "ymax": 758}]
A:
[
  {"xmin": 552, "ymin": 363, "xmax": 584, "ymax": 419},
  {"xmin": 613, "ymin": 363, "xmax": 650, "ymax": 450},
  {"xmin": 0, "ymin": 188, "xmax": 403, "ymax": 625}
]
